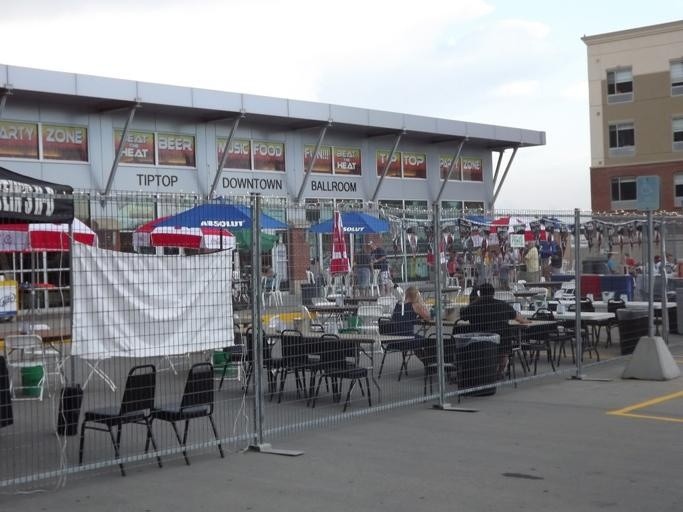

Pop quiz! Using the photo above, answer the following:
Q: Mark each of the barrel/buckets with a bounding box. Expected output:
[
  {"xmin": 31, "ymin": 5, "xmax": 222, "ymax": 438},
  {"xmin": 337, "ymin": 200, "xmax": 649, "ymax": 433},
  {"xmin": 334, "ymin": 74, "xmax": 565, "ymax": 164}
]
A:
[
  {"xmin": 348, "ymin": 316, "xmax": 359, "ymax": 328},
  {"xmin": 22, "ymin": 366, "xmax": 44, "ymax": 398},
  {"xmin": 213, "ymin": 351, "xmax": 226, "ymax": 365}
]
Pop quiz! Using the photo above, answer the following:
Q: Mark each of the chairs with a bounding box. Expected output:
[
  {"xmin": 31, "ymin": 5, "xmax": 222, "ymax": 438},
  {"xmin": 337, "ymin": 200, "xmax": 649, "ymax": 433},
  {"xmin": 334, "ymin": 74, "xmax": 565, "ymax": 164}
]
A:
[
  {"xmin": 298, "ymin": 280, "xmax": 329, "ymax": 325},
  {"xmin": 261, "ymin": 272, "xmax": 285, "ymax": 311},
  {"xmin": 3, "ymin": 333, "xmax": 51, "ymax": 402},
  {"xmin": 378, "ymin": 294, "xmax": 628, "ymax": 403},
  {"xmin": 218, "ymin": 321, "xmax": 372, "ymax": 413},
  {"xmin": 77, "ymin": 361, "xmax": 225, "ymax": 478}
]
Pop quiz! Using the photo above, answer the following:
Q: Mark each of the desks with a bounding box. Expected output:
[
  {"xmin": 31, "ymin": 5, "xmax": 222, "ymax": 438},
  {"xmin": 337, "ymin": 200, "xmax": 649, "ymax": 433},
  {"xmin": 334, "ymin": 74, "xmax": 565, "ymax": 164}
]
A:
[
  {"xmin": 417, "ymin": 287, "xmax": 461, "ymax": 306},
  {"xmin": 306, "ymin": 297, "xmax": 378, "ymax": 328},
  {"xmin": 234, "ymin": 329, "xmax": 416, "ymax": 405},
  {"xmin": 547, "ymin": 298, "xmax": 677, "ymax": 336}
]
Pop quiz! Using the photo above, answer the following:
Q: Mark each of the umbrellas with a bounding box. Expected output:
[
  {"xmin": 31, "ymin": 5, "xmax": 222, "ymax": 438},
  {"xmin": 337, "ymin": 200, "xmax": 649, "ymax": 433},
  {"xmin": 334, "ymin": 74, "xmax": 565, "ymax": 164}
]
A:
[{"xmin": 0, "ymin": 215, "xmax": 99, "ymax": 308}]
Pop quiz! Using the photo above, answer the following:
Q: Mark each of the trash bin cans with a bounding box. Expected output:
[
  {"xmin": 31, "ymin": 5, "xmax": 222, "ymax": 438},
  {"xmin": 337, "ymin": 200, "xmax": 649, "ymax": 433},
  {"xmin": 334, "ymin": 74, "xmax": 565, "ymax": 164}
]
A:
[
  {"xmin": 451, "ymin": 332, "xmax": 502, "ymax": 399},
  {"xmin": 616, "ymin": 307, "xmax": 649, "ymax": 356}
]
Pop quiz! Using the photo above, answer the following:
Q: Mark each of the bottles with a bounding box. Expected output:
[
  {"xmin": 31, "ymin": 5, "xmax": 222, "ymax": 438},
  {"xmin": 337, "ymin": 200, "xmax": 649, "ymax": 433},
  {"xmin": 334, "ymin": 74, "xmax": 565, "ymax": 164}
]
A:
[{"xmin": 272, "ymin": 232, "xmax": 288, "ymax": 281}]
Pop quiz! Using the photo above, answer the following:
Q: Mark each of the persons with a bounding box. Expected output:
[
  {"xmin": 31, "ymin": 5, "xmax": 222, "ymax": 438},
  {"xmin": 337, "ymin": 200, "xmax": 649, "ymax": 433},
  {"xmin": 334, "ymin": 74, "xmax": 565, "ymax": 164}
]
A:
[
  {"xmin": 460, "ymin": 284, "xmax": 529, "ymax": 380},
  {"xmin": 389, "ymin": 286, "xmax": 437, "ymax": 375},
  {"xmin": 607, "ymin": 252, "xmax": 678, "ymax": 275}
]
